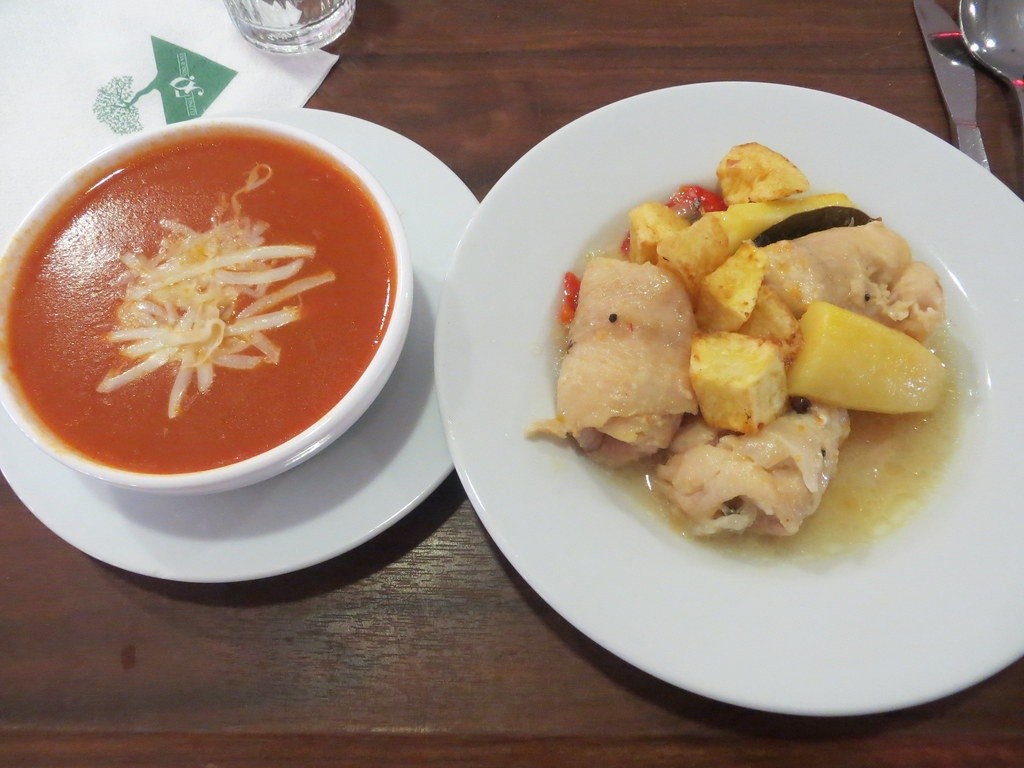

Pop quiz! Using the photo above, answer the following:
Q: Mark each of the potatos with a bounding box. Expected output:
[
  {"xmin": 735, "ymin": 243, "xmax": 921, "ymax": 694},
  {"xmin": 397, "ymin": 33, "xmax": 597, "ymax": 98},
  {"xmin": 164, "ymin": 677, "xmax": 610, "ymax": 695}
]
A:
[{"xmin": 625, "ymin": 142, "xmax": 944, "ymax": 434}]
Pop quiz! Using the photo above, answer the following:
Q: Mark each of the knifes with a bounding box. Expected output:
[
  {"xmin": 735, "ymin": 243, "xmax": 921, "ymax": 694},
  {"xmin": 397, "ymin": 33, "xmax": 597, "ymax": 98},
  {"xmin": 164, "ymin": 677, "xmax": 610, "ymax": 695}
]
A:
[{"xmin": 910, "ymin": 0, "xmax": 991, "ymax": 173}]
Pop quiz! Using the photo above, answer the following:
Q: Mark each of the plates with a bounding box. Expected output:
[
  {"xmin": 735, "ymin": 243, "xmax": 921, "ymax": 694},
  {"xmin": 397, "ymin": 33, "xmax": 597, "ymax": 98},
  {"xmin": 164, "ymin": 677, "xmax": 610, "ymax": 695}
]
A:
[
  {"xmin": 0, "ymin": 105, "xmax": 484, "ymax": 584},
  {"xmin": 430, "ymin": 79, "xmax": 1024, "ymax": 718}
]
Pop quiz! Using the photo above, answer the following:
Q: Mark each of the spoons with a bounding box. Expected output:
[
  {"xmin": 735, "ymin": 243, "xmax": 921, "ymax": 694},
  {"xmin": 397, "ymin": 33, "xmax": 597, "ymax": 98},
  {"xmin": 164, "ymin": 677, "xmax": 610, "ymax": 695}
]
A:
[{"xmin": 958, "ymin": 0, "xmax": 1024, "ymax": 133}]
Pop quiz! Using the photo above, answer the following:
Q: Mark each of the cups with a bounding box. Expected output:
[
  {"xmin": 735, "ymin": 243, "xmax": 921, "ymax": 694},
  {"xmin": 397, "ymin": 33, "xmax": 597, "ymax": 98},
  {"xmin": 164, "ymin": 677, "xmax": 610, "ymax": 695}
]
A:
[{"xmin": 222, "ymin": 0, "xmax": 357, "ymax": 54}]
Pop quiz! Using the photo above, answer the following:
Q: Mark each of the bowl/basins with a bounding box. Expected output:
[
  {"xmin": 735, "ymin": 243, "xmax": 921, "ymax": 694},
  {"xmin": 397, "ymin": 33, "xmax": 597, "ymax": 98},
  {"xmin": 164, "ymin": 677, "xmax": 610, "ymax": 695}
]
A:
[{"xmin": 1, "ymin": 117, "xmax": 414, "ymax": 497}]
